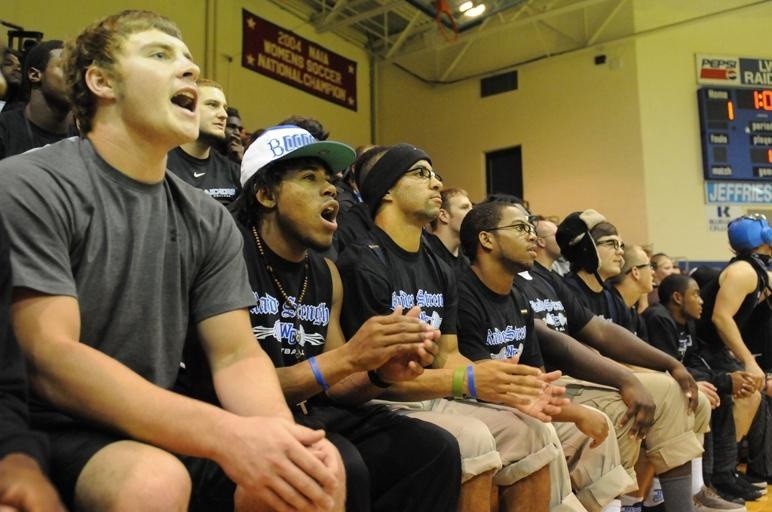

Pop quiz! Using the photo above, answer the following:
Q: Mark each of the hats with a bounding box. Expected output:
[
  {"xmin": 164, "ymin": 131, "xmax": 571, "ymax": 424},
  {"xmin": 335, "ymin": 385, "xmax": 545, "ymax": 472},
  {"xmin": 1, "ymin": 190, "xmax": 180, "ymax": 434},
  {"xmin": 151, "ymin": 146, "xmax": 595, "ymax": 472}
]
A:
[
  {"xmin": 554, "ymin": 208, "xmax": 607, "ymax": 275},
  {"xmin": 240, "ymin": 125, "xmax": 358, "ymax": 191}
]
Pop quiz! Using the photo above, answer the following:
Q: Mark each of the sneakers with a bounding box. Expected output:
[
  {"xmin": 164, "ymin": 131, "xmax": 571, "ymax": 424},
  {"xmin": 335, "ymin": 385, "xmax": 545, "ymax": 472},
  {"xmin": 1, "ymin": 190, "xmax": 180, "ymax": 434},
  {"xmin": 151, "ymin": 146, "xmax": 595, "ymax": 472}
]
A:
[{"xmin": 693, "ymin": 469, "xmax": 772, "ymax": 512}]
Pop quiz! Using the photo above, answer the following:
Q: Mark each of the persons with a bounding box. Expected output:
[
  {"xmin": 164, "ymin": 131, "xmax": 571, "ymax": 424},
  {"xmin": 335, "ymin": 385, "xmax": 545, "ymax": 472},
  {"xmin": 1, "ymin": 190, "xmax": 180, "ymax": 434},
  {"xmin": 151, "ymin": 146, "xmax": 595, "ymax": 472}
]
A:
[
  {"xmin": 432, "ymin": 185, "xmax": 772, "ymax": 512},
  {"xmin": 1, "ymin": 39, "xmax": 80, "ymax": 159},
  {"xmin": 2, "ymin": 8, "xmax": 345, "ymax": 511},
  {"xmin": 167, "ymin": 77, "xmax": 462, "ymax": 512},
  {"xmin": 336, "ymin": 142, "xmax": 567, "ymax": 512}
]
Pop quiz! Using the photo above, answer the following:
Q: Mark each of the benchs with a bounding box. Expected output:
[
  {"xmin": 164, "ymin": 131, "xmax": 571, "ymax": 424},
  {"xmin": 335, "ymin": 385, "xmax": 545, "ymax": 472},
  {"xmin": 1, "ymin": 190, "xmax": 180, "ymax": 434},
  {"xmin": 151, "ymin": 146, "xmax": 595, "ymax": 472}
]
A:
[{"xmin": 679, "ymin": 262, "xmax": 772, "ymax": 280}]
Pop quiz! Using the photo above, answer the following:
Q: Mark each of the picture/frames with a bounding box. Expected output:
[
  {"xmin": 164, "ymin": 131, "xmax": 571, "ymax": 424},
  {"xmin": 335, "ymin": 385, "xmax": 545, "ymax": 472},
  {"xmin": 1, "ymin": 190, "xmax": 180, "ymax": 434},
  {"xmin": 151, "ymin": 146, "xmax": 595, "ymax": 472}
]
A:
[{"xmin": 241, "ymin": 7, "xmax": 357, "ymax": 112}]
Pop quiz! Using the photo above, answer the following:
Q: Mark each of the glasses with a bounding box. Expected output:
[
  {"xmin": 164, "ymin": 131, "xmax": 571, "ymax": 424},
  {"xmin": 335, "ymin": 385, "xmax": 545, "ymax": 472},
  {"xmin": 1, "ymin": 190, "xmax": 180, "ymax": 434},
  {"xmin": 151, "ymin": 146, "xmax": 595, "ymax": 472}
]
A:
[
  {"xmin": 625, "ymin": 262, "xmax": 655, "ymax": 275},
  {"xmin": 596, "ymin": 239, "xmax": 625, "ymax": 251},
  {"xmin": 405, "ymin": 166, "xmax": 442, "ymax": 182},
  {"xmin": 489, "ymin": 224, "xmax": 538, "ymax": 237}
]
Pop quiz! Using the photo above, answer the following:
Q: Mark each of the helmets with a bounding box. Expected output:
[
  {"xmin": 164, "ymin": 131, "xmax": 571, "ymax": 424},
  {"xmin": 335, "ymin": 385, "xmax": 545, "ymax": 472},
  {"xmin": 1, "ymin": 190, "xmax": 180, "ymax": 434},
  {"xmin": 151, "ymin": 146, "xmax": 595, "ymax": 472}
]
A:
[{"xmin": 727, "ymin": 213, "xmax": 772, "ymax": 253}]
